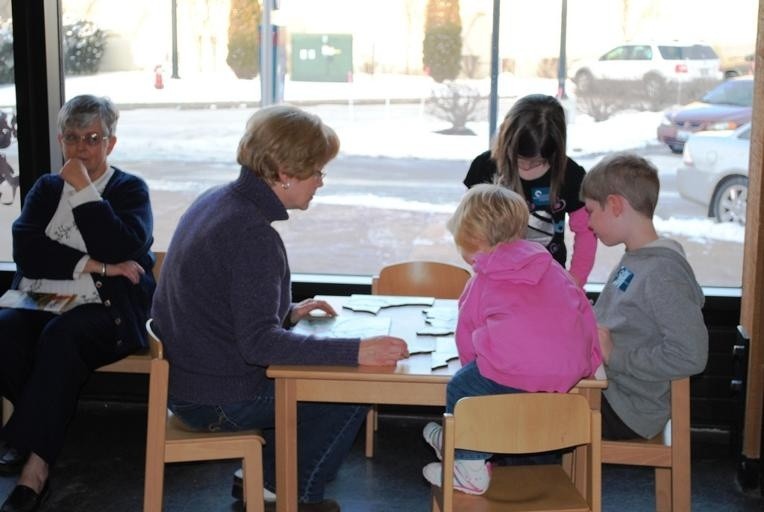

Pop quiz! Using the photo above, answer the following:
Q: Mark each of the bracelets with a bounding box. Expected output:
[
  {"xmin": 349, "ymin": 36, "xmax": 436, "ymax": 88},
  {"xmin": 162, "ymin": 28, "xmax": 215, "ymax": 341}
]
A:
[{"xmin": 100, "ymin": 262, "xmax": 106, "ymax": 278}]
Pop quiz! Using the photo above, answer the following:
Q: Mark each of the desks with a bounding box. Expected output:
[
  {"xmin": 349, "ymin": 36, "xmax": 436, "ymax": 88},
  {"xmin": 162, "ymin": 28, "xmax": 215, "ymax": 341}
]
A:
[{"xmin": 265, "ymin": 294, "xmax": 608, "ymax": 512}]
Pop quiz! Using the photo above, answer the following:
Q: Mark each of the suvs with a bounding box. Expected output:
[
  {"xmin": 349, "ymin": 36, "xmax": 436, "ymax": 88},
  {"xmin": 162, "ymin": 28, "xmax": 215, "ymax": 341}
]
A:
[{"xmin": 568, "ymin": 35, "xmax": 727, "ymax": 96}]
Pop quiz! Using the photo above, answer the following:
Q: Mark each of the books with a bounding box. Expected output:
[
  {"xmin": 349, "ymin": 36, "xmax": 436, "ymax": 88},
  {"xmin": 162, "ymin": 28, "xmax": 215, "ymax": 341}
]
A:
[{"xmin": 0, "ymin": 289, "xmax": 77, "ymax": 314}]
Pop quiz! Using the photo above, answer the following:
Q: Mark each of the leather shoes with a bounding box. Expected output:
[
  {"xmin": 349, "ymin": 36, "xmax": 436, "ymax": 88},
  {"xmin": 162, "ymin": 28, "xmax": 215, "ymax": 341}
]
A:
[{"xmin": 0, "ymin": 448, "xmax": 27, "ymax": 474}]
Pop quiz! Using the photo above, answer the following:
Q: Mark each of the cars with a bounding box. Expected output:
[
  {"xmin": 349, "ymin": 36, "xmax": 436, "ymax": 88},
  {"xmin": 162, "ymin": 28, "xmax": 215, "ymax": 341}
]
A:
[
  {"xmin": 721, "ymin": 54, "xmax": 754, "ymax": 80},
  {"xmin": 666, "ymin": 116, "xmax": 754, "ymax": 226},
  {"xmin": 647, "ymin": 74, "xmax": 757, "ymax": 155}
]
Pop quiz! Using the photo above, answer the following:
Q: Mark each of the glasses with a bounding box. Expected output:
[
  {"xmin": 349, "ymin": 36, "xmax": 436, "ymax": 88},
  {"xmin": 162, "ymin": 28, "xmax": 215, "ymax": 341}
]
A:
[{"xmin": 61, "ymin": 131, "xmax": 110, "ymax": 146}]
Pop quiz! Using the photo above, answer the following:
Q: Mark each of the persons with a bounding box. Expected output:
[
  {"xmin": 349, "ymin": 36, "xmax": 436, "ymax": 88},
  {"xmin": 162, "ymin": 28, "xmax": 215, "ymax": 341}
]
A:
[
  {"xmin": 0, "ymin": 96, "xmax": 156, "ymax": 512},
  {"xmin": 482, "ymin": 152, "xmax": 709, "ymax": 467},
  {"xmin": 422, "ymin": 182, "xmax": 600, "ymax": 495},
  {"xmin": 152, "ymin": 106, "xmax": 411, "ymax": 511},
  {"xmin": 463, "ymin": 95, "xmax": 598, "ymax": 292}
]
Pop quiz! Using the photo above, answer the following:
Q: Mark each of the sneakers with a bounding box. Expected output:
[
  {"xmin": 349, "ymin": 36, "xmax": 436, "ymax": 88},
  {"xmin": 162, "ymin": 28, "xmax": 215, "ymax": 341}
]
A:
[
  {"xmin": 423, "ymin": 420, "xmax": 444, "ymax": 460},
  {"xmin": 0, "ymin": 478, "xmax": 50, "ymax": 511},
  {"xmin": 422, "ymin": 460, "xmax": 491, "ymax": 496},
  {"xmin": 230, "ymin": 469, "xmax": 339, "ymax": 512}
]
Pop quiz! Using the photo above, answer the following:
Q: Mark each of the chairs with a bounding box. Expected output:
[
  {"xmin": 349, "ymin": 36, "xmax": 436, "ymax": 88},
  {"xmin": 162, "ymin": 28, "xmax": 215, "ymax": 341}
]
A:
[
  {"xmin": 430, "ymin": 392, "xmax": 602, "ymax": 512},
  {"xmin": 602, "ymin": 377, "xmax": 691, "ymax": 512},
  {"xmin": 365, "ymin": 260, "xmax": 473, "ymax": 459},
  {"xmin": 142, "ymin": 316, "xmax": 267, "ymax": 512},
  {"xmin": 3, "ymin": 251, "xmax": 169, "ymax": 426}
]
